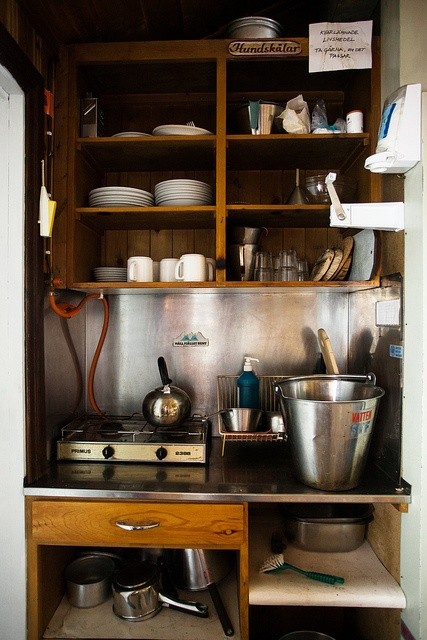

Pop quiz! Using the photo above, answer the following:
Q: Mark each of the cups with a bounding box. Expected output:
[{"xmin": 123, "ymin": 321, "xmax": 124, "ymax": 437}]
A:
[
  {"xmin": 175, "ymin": 254, "xmax": 209, "ymax": 281},
  {"xmin": 126, "ymin": 255, "xmax": 155, "ymax": 283},
  {"xmin": 254, "ymin": 249, "xmax": 310, "ymax": 281},
  {"xmin": 160, "ymin": 258, "xmax": 179, "ymax": 281}
]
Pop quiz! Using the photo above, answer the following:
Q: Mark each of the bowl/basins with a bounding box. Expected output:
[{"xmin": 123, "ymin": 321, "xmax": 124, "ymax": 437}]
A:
[
  {"xmin": 270, "ymin": 414, "xmax": 286, "ymax": 431},
  {"xmin": 64, "ymin": 555, "xmax": 108, "ymax": 607},
  {"xmin": 230, "ymin": 15, "xmax": 278, "ymax": 41},
  {"xmin": 238, "ymin": 225, "xmax": 268, "ymax": 243},
  {"xmin": 291, "ymin": 517, "xmax": 367, "ymax": 554},
  {"xmin": 299, "ymin": 177, "xmax": 343, "ymax": 202}
]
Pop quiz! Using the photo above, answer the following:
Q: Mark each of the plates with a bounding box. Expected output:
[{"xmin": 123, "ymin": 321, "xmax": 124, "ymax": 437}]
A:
[
  {"xmin": 153, "ymin": 125, "xmax": 211, "ymax": 134},
  {"xmin": 92, "ymin": 266, "xmax": 126, "ymax": 282},
  {"xmin": 153, "ymin": 178, "xmax": 211, "ymax": 206},
  {"xmin": 87, "ymin": 185, "xmax": 153, "ymax": 206},
  {"xmin": 113, "ymin": 132, "xmax": 151, "ymax": 136}
]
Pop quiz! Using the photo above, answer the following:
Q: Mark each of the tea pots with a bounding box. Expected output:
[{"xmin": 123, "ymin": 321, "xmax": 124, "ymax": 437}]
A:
[
  {"xmin": 142, "ymin": 356, "xmax": 191, "ymax": 427},
  {"xmin": 233, "ymin": 244, "xmax": 256, "ymax": 280}
]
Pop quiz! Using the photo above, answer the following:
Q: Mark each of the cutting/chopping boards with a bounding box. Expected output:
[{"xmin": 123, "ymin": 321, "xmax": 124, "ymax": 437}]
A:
[{"xmin": 350, "ymin": 228, "xmax": 375, "ymax": 283}]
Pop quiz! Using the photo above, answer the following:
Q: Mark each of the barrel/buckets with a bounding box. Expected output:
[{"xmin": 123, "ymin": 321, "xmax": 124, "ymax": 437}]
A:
[{"xmin": 274, "ymin": 372, "xmax": 385, "ymax": 494}]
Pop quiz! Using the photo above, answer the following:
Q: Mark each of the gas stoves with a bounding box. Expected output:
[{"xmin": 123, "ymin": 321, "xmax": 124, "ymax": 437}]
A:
[{"xmin": 57, "ymin": 417, "xmax": 207, "ymax": 463}]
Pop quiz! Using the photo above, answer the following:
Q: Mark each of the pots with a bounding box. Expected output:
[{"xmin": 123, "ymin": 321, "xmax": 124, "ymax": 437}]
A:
[
  {"xmin": 112, "ymin": 562, "xmax": 208, "ymax": 623},
  {"xmin": 202, "ymin": 407, "xmax": 262, "ymax": 431},
  {"xmin": 172, "ymin": 548, "xmax": 235, "ymax": 635}
]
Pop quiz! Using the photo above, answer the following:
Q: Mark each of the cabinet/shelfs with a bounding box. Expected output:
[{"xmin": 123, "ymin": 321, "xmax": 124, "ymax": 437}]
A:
[
  {"xmin": 47, "ymin": 6, "xmax": 380, "ymax": 291},
  {"xmin": 24, "ymin": 437, "xmax": 413, "ymax": 629}
]
